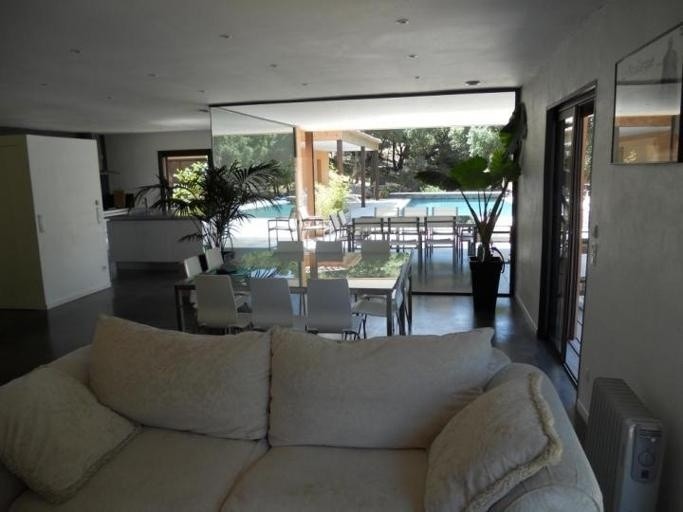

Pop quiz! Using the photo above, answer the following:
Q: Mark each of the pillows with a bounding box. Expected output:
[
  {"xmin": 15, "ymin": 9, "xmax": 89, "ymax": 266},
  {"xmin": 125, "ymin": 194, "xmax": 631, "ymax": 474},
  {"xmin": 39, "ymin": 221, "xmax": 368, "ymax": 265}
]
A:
[
  {"xmin": 0, "ymin": 365, "xmax": 143, "ymax": 504},
  {"xmin": 423, "ymin": 371, "xmax": 563, "ymax": 511},
  {"xmin": 269, "ymin": 324, "xmax": 495, "ymax": 450},
  {"xmin": 89, "ymin": 314, "xmax": 270, "ymax": 440}
]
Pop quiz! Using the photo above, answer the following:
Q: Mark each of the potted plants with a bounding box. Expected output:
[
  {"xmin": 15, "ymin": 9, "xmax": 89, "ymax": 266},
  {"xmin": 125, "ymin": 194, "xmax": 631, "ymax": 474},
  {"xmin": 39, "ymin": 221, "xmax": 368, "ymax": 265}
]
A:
[
  {"xmin": 128, "ymin": 159, "xmax": 289, "ymax": 268},
  {"xmin": 414, "ymin": 148, "xmax": 514, "ymax": 316}
]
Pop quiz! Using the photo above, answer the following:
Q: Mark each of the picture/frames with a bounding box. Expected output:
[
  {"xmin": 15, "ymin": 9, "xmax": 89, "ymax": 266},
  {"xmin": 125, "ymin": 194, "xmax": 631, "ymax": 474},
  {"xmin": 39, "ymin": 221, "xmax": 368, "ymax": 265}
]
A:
[{"xmin": 610, "ymin": 21, "xmax": 683, "ymax": 166}]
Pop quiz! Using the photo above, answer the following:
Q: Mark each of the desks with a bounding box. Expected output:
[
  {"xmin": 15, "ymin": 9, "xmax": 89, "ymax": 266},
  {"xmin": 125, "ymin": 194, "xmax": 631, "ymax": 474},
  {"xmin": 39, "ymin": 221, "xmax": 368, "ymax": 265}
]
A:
[
  {"xmin": 346, "ymin": 216, "xmax": 469, "ymax": 259},
  {"xmin": 174, "ymin": 252, "xmax": 413, "ymax": 337}
]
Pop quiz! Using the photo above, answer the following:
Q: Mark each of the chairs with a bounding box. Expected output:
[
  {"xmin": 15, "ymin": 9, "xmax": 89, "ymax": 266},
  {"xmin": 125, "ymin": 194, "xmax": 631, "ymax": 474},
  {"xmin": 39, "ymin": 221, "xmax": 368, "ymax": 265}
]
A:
[
  {"xmin": 205, "ymin": 248, "xmax": 253, "ymax": 310},
  {"xmin": 183, "ymin": 256, "xmax": 247, "ymax": 333},
  {"xmin": 249, "ymin": 277, "xmax": 300, "ymax": 331},
  {"xmin": 402, "ymin": 207, "xmax": 428, "ymax": 248},
  {"xmin": 361, "ymin": 251, "xmax": 414, "ymax": 334},
  {"xmin": 387, "ymin": 217, "xmax": 421, "ymax": 271},
  {"xmin": 297, "ymin": 200, "xmax": 331, "ymax": 251},
  {"xmin": 370, "ymin": 206, "xmax": 399, "ymax": 244},
  {"xmin": 338, "ymin": 211, "xmax": 355, "ymax": 238},
  {"xmin": 432, "ymin": 207, "xmax": 459, "ymax": 249},
  {"xmin": 329, "ymin": 212, "xmax": 355, "ymax": 247},
  {"xmin": 353, "ymin": 260, "xmax": 406, "ymax": 336},
  {"xmin": 277, "ymin": 240, "xmax": 304, "ymax": 253},
  {"xmin": 361, "ymin": 240, "xmax": 391, "ymax": 254},
  {"xmin": 193, "ymin": 274, "xmax": 251, "ymax": 335},
  {"xmin": 352, "ymin": 217, "xmax": 384, "ymax": 251},
  {"xmin": 267, "ymin": 203, "xmax": 296, "ymax": 251},
  {"xmin": 424, "ymin": 216, "xmax": 459, "ymax": 273},
  {"xmin": 315, "ymin": 240, "xmax": 343, "ymax": 254},
  {"xmin": 456, "ymin": 217, "xmax": 478, "ymax": 258},
  {"xmin": 306, "ymin": 278, "xmax": 366, "ymax": 341}
]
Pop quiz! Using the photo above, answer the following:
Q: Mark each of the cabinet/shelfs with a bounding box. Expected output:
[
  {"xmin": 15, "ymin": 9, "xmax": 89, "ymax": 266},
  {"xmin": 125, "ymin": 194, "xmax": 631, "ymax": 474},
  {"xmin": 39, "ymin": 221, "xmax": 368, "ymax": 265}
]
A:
[{"xmin": 103, "ymin": 208, "xmax": 221, "ymax": 271}]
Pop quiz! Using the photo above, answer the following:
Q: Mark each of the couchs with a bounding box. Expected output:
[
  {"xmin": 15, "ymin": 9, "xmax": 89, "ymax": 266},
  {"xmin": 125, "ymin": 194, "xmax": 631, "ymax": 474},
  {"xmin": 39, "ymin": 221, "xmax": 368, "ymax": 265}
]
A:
[{"xmin": 0, "ymin": 313, "xmax": 605, "ymax": 511}]
